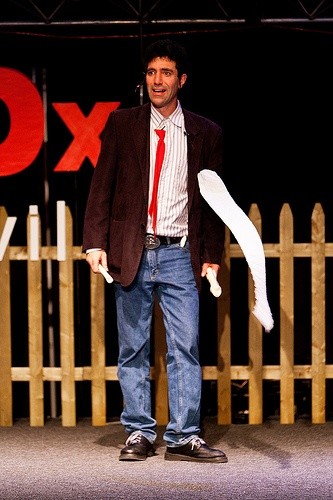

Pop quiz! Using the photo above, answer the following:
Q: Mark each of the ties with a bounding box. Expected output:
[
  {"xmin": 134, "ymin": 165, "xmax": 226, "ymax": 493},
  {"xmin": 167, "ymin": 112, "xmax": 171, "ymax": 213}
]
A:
[{"xmin": 149, "ymin": 129, "xmax": 165, "ymax": 243}]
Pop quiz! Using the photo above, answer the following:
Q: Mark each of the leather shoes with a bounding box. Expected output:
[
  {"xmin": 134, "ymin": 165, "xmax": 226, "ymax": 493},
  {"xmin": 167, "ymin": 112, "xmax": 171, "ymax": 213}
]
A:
[
  {"xmin": 164, "ymin": 437, "xmax": 228, "ymax": 463},
  {"xmin": 119, "ymin": 430, "xmax": 157, "ymax": 460}
]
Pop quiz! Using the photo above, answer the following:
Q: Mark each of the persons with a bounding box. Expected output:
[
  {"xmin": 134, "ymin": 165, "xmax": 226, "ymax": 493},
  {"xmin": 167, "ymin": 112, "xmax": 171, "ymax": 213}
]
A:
[{"xmin": 80, "ymin": 44, "xmax": 230, "ymax": 461}]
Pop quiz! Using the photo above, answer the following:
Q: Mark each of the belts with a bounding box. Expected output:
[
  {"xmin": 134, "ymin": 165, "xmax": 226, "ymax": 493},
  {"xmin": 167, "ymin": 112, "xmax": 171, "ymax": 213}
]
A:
[{"xmin": 144, "ymin": 233, "xmax": 189, "ymax": 249}]
